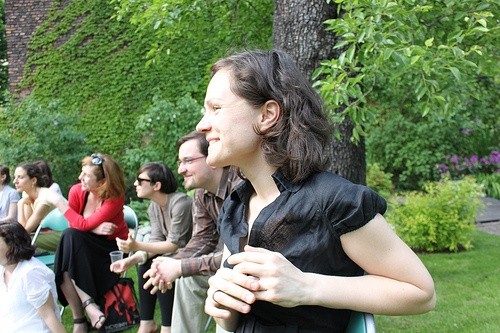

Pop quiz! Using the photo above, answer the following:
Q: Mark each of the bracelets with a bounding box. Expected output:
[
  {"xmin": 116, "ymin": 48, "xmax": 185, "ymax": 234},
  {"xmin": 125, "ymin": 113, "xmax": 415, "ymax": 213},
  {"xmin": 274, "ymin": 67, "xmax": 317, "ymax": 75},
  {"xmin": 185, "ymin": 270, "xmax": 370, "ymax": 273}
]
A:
[{"xmin": 136, "ymin": 251, "xmax": 149, "ymax": 266}]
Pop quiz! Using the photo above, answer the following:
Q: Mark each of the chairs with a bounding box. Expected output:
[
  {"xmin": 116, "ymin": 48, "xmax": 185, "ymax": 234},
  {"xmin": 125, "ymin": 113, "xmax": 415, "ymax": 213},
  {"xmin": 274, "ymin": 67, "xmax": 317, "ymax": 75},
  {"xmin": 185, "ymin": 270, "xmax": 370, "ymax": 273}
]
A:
[{"xmin": 31, "ymin": 205, "xmax": 138, "ymax": 316}]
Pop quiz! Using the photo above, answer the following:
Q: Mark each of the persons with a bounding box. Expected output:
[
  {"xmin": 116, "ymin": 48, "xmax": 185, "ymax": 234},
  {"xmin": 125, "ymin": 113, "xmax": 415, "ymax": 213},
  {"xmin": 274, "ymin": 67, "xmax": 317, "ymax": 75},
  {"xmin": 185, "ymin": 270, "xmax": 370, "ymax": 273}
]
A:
[
  {"xmin": 0, "ymin": 160, "xmax": 68, "ymax": 333},
  {"xmin": 108, "ymin": 160, "xmax": 192, "ymax": 333},
  {"xmin": 196, "ymin": 51, "xmax": 436, "ymax": 333},
  {"xmin": 142, "ymin": 131, "xmax": 231, "ymax": 333},
  {"xmin": 46, "ymin": 153, "xmax": 130, "ymax": 333}
]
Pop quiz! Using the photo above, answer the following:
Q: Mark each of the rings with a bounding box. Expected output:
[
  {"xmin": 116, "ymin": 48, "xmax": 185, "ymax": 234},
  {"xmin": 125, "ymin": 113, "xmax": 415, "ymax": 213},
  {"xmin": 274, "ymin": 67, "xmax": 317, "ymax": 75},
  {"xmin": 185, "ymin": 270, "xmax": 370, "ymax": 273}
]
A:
[{"xmin": 212, "ymin": 289, "xmax": 221, "ymax": 302}]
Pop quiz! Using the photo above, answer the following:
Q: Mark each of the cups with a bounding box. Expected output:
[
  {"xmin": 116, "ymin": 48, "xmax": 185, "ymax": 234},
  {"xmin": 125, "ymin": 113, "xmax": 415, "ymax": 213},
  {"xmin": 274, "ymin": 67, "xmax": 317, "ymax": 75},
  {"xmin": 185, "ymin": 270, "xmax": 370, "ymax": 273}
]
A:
[{"xmin": 109, "ymin": 251, "xmax": 123, "ymax": 272}]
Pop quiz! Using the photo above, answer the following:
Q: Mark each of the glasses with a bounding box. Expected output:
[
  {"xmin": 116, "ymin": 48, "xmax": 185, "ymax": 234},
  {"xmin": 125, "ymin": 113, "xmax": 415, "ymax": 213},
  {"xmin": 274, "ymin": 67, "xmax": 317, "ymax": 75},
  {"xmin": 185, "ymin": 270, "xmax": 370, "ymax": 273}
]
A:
[
  {"xmin": 137, "ymin": 176, "xmax": 156, "ymax": 185},
  {"xmin": 176, "ymin": 155, "xmax": 207, "ymax": 168},
  {"xmin": 91, "ymin": 153, "xmax": 105, "ymax": 178}
]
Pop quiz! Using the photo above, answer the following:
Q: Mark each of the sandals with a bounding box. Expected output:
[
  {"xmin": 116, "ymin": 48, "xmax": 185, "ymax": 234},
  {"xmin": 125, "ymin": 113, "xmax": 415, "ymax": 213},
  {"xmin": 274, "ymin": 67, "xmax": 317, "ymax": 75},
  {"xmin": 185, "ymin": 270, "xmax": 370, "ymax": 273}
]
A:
[
  {"xmin": 73, "ymin": 316, "xmax": 92, "ymax": 333},
  {"xmin": 82, "ymin": 298, "xmax": 106, "ymax": 330}
]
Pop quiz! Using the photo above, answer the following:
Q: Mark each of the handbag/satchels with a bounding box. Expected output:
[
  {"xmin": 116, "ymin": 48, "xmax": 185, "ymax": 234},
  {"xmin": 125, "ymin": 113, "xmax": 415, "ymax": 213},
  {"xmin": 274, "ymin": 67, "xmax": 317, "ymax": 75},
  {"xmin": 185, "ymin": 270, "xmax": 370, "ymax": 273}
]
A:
[{"xmin": 104, "ymin": 278, "xmax": 141, "ymax": 333}]
